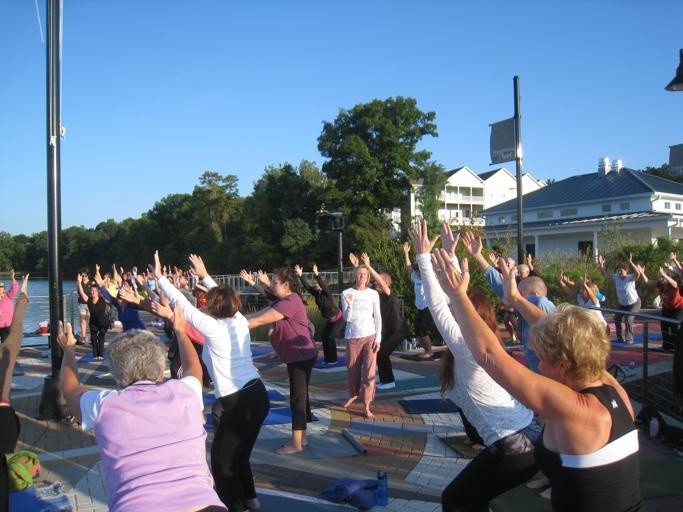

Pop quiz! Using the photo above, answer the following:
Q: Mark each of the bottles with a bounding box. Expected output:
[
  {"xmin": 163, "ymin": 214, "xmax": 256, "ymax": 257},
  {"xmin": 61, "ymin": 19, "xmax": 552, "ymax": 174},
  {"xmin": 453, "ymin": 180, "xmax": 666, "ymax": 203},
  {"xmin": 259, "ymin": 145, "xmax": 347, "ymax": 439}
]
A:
[
  {"xmin": 628, "ymin": 358, "xmax": 635, "ymax": 372},
  {"xmin": 649, "ymin": 417, "xmax": 658, "ymax": 437},
  {"xmin": 401, "ymin": 336, "xmax": 416, "ymax": 354},
  {"xmin": 374, "ymin": 469, "xmax": 388, "ymax": 506}
]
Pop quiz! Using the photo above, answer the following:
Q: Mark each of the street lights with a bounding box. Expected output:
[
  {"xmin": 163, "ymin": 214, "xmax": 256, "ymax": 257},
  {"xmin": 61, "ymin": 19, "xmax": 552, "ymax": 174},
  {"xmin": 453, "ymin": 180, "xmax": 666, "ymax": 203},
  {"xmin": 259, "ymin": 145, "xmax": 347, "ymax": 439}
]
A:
[
  {"xmin": 666, "ymin": 47, "xmax": 683, "ymax": 94},
  {"xmin": 326, "ymin": 210, "xmax": 345, "ymax": 316}
]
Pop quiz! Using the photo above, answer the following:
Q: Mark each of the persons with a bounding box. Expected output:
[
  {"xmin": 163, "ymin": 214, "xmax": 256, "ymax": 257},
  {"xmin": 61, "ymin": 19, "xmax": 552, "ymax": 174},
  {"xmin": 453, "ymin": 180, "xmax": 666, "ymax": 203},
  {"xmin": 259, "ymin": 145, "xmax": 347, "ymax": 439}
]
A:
[{"xmin": 1, "ymin": 217, "xmax": 683, "ymax": 511}]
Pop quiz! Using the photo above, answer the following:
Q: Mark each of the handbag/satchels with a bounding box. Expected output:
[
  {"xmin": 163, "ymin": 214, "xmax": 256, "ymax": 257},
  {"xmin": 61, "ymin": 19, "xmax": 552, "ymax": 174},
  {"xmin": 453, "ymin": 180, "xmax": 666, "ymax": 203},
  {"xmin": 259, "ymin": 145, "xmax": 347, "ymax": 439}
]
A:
[{"xmin": 4, "ymin": 449, "xmax": 40, "ymax": 492}]
